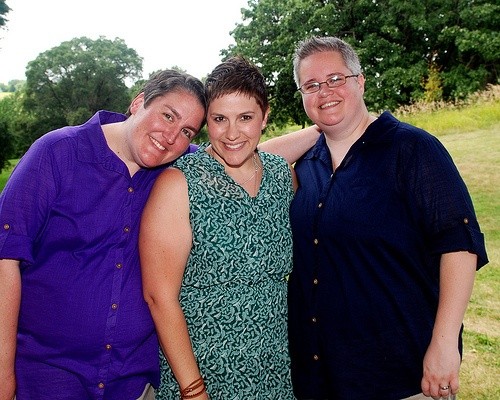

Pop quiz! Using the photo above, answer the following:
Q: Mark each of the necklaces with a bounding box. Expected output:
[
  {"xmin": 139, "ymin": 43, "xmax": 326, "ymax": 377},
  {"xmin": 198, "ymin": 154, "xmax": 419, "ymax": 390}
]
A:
[{"xmin": 209, "ymin": 150, "xmax": 259, "ymax": 197}]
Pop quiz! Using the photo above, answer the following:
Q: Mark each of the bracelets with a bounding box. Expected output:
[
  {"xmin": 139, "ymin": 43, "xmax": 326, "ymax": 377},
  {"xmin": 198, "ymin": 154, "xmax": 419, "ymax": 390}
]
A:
[{"xmin": 180, "ymin": 377, "xmax": 207, "ymax": 398}]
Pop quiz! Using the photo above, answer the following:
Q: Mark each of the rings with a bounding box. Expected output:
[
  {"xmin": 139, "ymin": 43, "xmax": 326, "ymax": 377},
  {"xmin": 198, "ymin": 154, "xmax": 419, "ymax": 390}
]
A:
[{"xmin": 440, "ymin": 385, "xmax": 449, "ymax": 390}]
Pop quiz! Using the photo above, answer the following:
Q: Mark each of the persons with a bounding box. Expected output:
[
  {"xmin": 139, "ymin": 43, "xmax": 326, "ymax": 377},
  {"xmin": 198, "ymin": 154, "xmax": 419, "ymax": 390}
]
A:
[
  {"xmin": 138, "ymin": 55, "xmax": 298, "ymax": 400},
  {"xmin": 0, "ymin": 70, "xmax": 322, "ymax": 400},
  {"xmin": 288, "ymin": 35, "xmax": 489, "ymax": 400}
]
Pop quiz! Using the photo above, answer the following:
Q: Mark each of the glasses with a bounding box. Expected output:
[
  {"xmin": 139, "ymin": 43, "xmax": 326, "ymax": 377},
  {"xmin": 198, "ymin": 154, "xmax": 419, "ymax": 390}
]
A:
[{"xmin": 297, "ymin": 74, "xmax": 359, "ymax": 94}]
